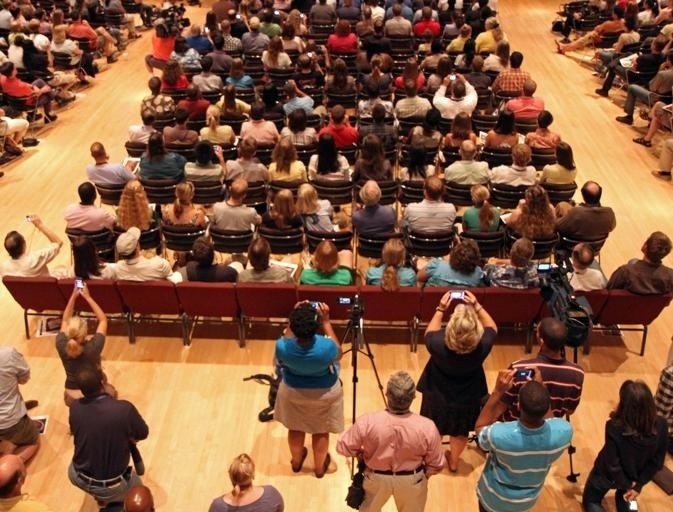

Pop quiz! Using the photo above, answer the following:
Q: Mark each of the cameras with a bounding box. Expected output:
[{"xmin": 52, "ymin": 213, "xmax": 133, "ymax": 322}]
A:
[
  {"xmin": 450, "ymin": 289, "xmax": 465, "ymax": 300},
  {"xmin": 25, "ymin": 216, "xmax": 32, "ymax": 221},
  {"xmin": 309, "ymin": 301, "xmax": 320, "ymax": 311},
  {"xmin": 337, "ymin": 294, "xmax": 364, "ymax": 326},
  {"xmin": 514, "ymin": 369, "xmax": 535, "ymax": 380},
  {"xmin": 346, "ymin": 473, "xmax": 365, "ymax": 510}
]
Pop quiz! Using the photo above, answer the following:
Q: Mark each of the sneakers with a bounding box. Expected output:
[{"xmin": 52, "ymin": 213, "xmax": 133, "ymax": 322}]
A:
[
  {"xmin": 596, "ymin": 88, "xmax": 608, "ymax": 96},
  {"xmin": 44, "ymin": 115, "xmax": 56, "ymax": 123},
  {"xmin": 316, "ymin": 453, "xmax": 330, "ymax": 477},
  {"xmin": 651, "ymin": 170, "xmax": 671, "ymax": 182},
  {"xmin": 27, "ymin": 113, "xmax": 42, "ymax": 122},
  {"xmin": 293, "ymin": 447, "xmax": 307, "ymax": 472},
  {"xmin": 616, "ymin": 116, "xmax": 633, "ymax": 125}
]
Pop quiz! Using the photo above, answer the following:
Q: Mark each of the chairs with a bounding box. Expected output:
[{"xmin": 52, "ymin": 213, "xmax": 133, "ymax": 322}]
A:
[{"xmin": 0, "ymin": 0, "xmax": 673, "ymax": 357}]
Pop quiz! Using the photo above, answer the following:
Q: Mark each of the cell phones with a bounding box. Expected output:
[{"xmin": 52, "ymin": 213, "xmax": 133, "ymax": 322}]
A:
[{"xmin": 74, "ymin": 277, "xmax": 84, "ymax": 292}]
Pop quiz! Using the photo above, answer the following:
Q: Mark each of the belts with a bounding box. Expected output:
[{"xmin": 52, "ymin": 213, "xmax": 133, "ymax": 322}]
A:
[
  {"xmin": 78, "ymin": 474, "xmax": 125, "ymax": 487},
  {"xmin": 374, "ymin": 466, "xmax": 423, "ymax": 476}
]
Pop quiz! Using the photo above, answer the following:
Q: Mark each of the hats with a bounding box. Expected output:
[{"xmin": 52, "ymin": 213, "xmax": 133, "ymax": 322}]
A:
[{"xmin": 116, "ymin": 227, "xmax": 141, "ymax": 256}]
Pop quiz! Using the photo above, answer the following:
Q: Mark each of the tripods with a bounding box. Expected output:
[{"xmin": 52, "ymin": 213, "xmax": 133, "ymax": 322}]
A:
[{"xmin": 340, "ymin": 318, "xmax": 388, "ymax": 480}]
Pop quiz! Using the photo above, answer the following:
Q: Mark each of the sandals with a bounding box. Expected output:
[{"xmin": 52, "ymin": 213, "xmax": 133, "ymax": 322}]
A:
[{"xmin": 633, "ymin": 138, "xmax": 651, "ymax": 146}]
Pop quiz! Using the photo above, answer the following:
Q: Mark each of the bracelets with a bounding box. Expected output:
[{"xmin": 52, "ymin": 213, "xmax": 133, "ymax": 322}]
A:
[
  {"xmin": 476, "ymin": 305, "xmax": 482, "ymax": 312},
  {"xmin": 437, "ymin": 308, "xmax": 446, "ymax": 313}
]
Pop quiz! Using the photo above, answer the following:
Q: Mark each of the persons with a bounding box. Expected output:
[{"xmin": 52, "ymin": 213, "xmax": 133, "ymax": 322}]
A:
[
  {"xmin": 0, "ymin": 346, "xmax": 54, "ymax": 512},
  {"xmin": 68, "ymin": 363, "xmax": 149, "ymax": 512},
  {"xmin": 582, "ymin": 365, "xmax": 673, "ymax": 512},
  {"xmin": 554, "ymin": 0, "xmax": 673, "ymax": 177},
  {"xmin": 475, "ymin": 366, "xmax": 573, "ymax": 512},
  {"xmin": 124, "ymin": 485, "xmax": 155, "ymax": 512},
  {"xmin": 609, "ymin": 232, "xmax": 671, "ymax": 295},
  {"xmin": 416, "ymin": 291, "xmax": 497, "ymax": 473},
  {"xmin": 208, "ymin": 453, "xmax": 284, "ymax": 512},
  {"xmin": 336, "ymin": 371, "xmax": 446, "ymax": 512},
  {"xmin": 273, "ymin": 300, "xmax": 345, "ymax": 478},
  {"xmin": 0, "ymin": 0, "xmax": 616, "ymax": 342},
  {"xmin": 493, "ymin": 319, "xmax": 585, "ymax": 423},
  {"xmin": 55, "ymin": 282, "xmax": 117, "ymax": 406}
]
